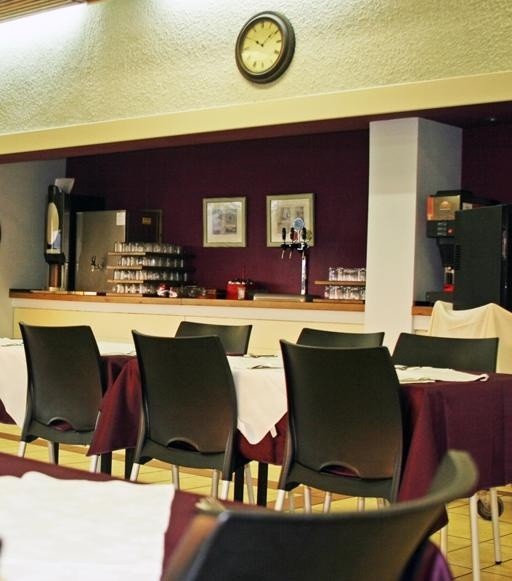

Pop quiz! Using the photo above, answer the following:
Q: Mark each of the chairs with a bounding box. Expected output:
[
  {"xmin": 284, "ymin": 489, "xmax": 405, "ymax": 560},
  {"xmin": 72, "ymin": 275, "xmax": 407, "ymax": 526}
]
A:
[
  {"xmin": 389, "ymin": 329, "xmax": 501, "ymax": 374},
  {"xmin": 273, "ymin": 336, "xmax": 413, "ymax": 517},
  {"xmin": 173, "ymin": 320, "xmax": 254, "ymax": 357},
  {"xmin": 289, "ymin": 326, "xmax": 386, "ymax": 348},
  {"xmin": 185, "ymin": 449, "xmax": 483, "ymax": 578},
  {"xmin": 17, "ymin": 320, "xmax": 110, "ymax": 474},
  {"xmin": 126, "ymin": 326, "xmax": 257, "ymax": 510}
]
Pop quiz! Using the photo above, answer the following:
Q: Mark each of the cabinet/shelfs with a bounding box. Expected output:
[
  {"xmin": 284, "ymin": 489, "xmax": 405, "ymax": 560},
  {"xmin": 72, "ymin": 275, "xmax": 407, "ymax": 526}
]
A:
[
  {"xmin": 312, "ymin": 279, "xmax": 366, "ymax": 303},
  {"xmin": 106, "ymin": 251, "xmax": 196, "ymax": 297}
]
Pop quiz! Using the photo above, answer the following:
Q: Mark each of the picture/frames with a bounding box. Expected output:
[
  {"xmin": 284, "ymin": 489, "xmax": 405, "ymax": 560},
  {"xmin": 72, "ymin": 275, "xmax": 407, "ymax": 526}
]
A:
[
  {"xmin": 201, "ymin": 195, "xmax": 247, "ymax": 248},
  {"xmin": 266, "ymin": 192, "xmax": 315, "ymax": 247}
]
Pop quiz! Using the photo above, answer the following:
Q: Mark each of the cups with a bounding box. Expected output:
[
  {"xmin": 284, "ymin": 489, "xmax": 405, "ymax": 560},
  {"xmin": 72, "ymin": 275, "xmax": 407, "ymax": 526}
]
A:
[
  {"xmin": 111, "ymin": 241, "xmax": 187, "ymax": 294},
  {"xmin": 324, "ymin": 267, "xmax": 366, "ymax": 300}
]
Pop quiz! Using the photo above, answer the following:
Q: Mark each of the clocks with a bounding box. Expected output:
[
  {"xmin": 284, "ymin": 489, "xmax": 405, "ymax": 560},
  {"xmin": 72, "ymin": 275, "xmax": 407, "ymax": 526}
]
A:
[{"xmin": 234, "ymin": 10, "xmax": 296, "ymax": 83}]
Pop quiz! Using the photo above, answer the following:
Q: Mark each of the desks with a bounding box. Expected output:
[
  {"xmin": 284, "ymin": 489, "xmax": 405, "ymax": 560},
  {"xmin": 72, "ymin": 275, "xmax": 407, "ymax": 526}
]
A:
[
  {"xmin": 0, "ymin": 334, "xmax": 512, "ymax": 564},
  {"xmin": 0, "ymin": 452, "xmax": 275, "ymax": 580}
]
